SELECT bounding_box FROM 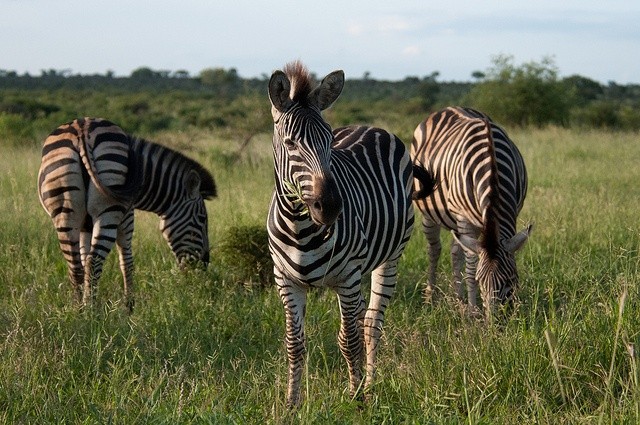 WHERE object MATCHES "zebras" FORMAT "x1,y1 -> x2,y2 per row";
37,117 -> 218,321
267,62 -> 443,415
408,105 -> 532,334
